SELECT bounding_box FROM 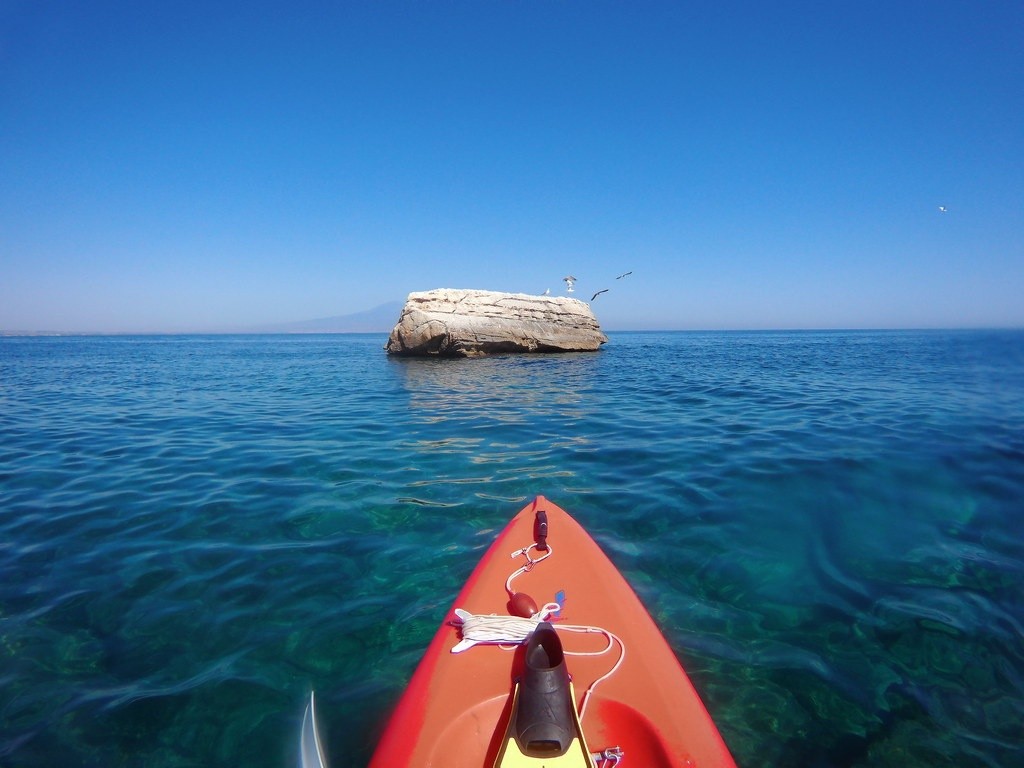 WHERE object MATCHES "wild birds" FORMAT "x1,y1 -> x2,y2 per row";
591,289 -> 609,301
616,271 -> 633,279
541,288 -> 550,296
939,206 -> 947,213
